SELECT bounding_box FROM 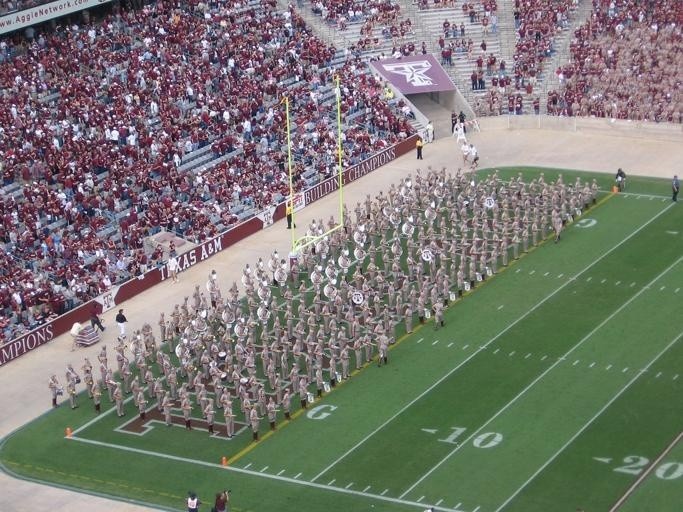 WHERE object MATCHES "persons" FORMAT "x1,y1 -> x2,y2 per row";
671,175 -> 679,202
616,169 -> 626,192
601,1 -> 683,126
184,491 -> 202,512
214,489 -> 231,512
0,1 -> 600,443
